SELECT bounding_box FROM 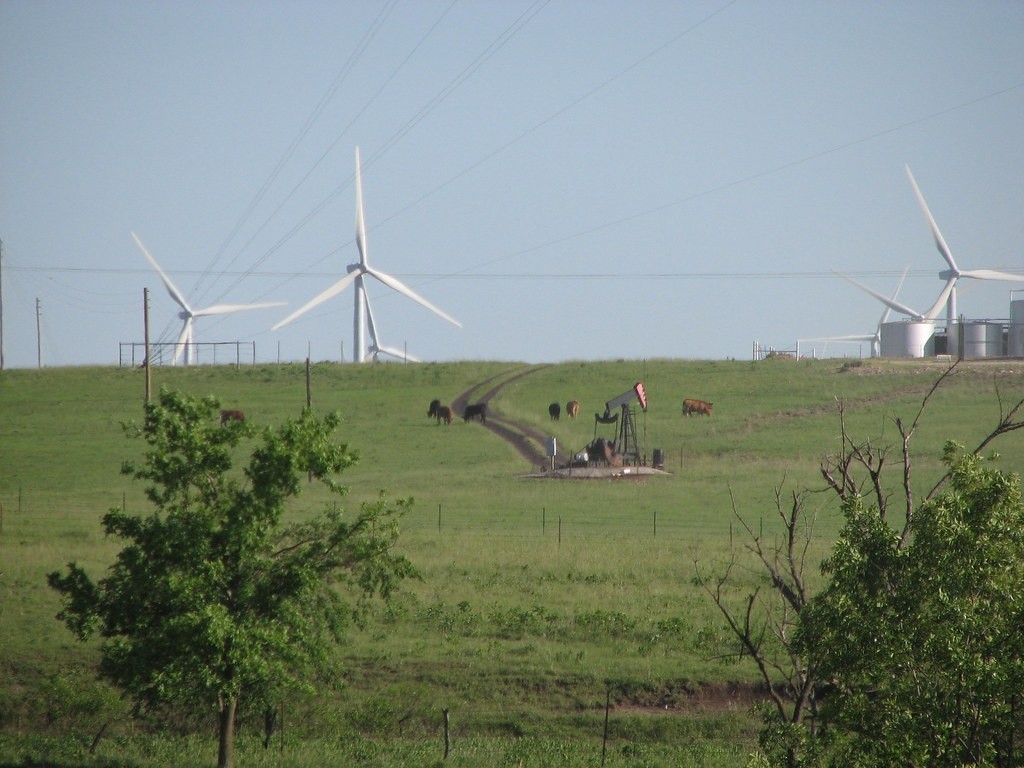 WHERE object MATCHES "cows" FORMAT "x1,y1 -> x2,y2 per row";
427,400 -> 453,425
566,400 -> 580,418
682,398 -> 714,418
462,403 -> 486,424
549,404 -> 560,421
